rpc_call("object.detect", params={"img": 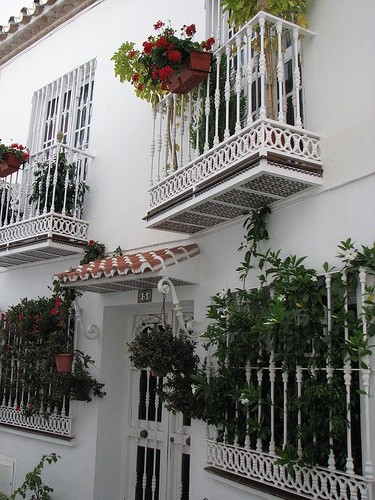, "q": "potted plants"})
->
[111,18,215,97]
[125,295,173,379]
[28,334,104,403]
[0,138,32,176]
